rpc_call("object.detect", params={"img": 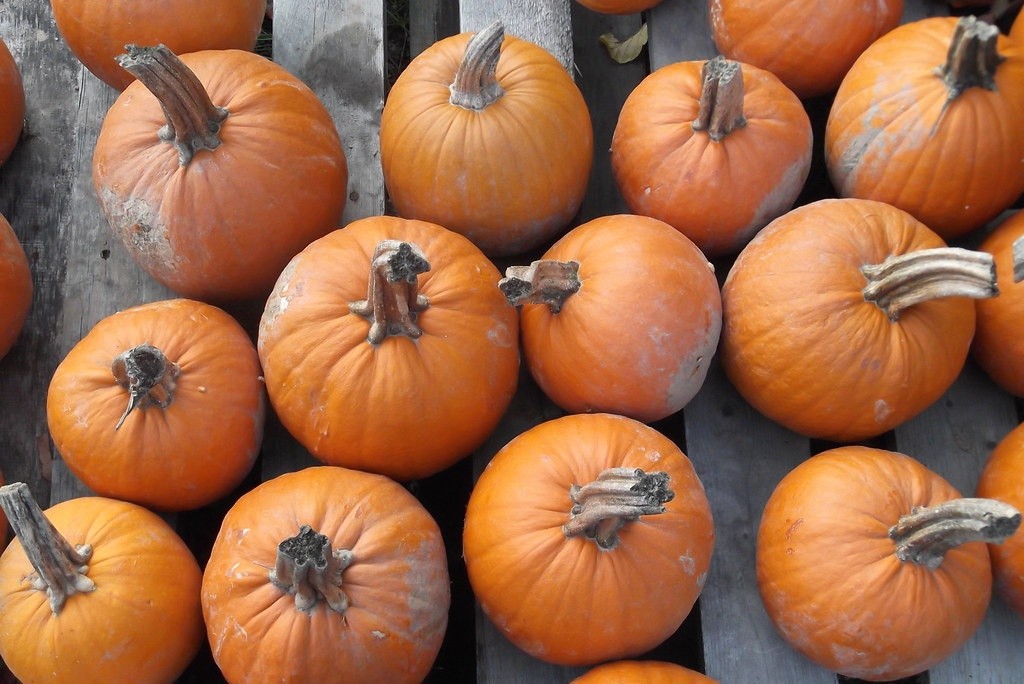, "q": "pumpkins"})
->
[0,0,1024,684]
